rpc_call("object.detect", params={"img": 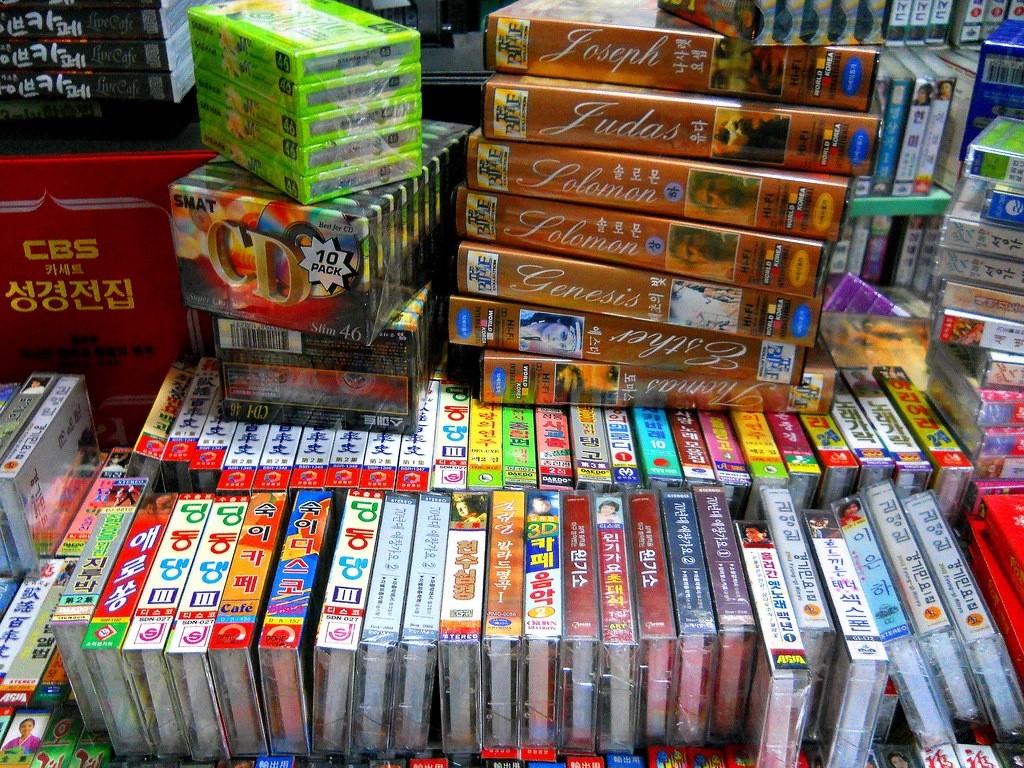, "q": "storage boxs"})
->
[0,0,1024,768]
